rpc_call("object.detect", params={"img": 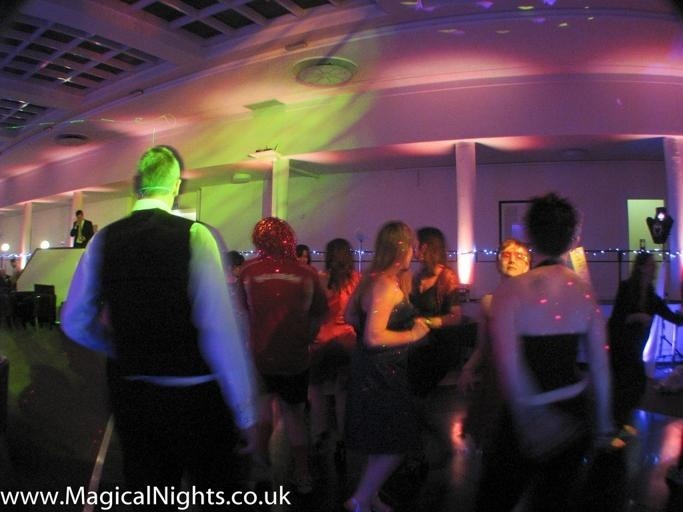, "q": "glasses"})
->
[499,252,522,261]
